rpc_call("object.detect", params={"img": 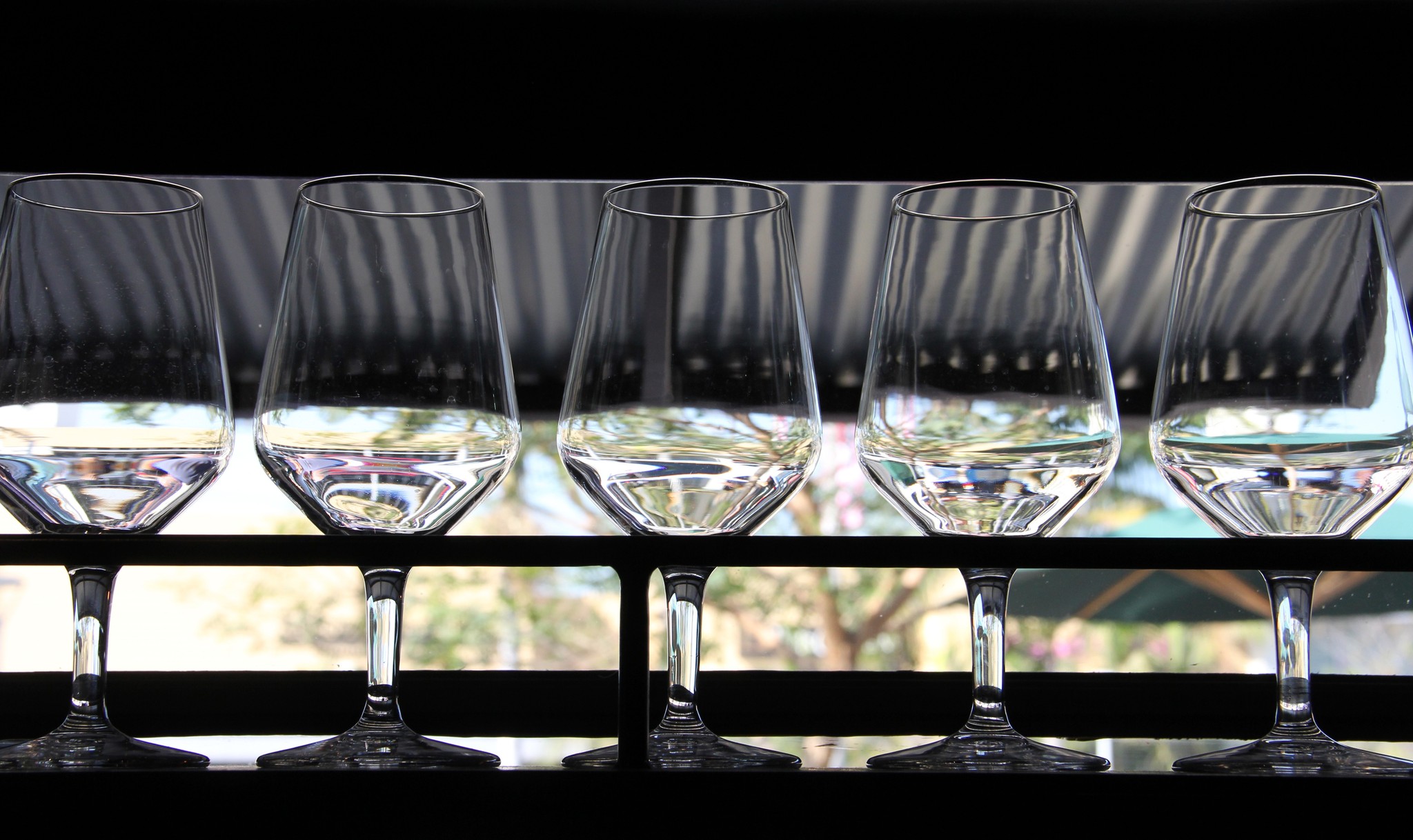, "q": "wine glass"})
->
[556,177,822,772]
[1148,171,1413,777]
[252,174,523,773]
[0,170,233,772]
[851,176,1121,773]
